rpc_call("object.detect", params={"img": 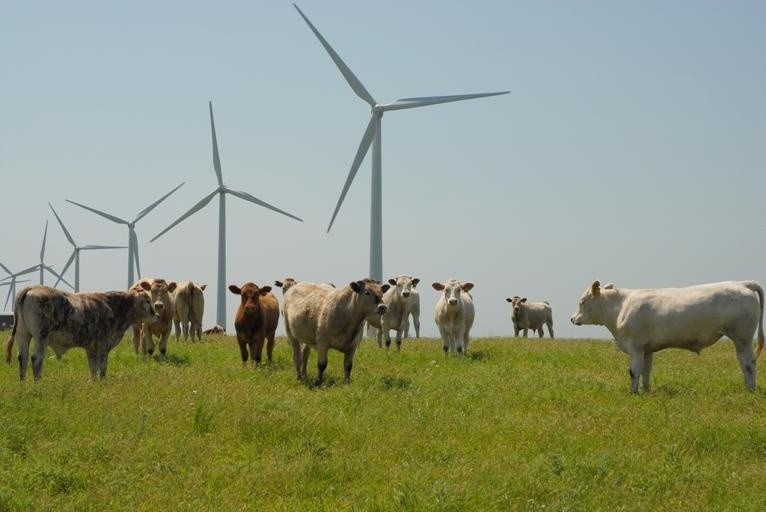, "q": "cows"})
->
[432,277,475,359]
[127,277,177,364]
[274,275,421,390]
[5,283,160,384]
[228,283,280,368]
[202,325,226,335]
[170,280,208,345]
[506,296,553,338]
[571,281,765,394]
